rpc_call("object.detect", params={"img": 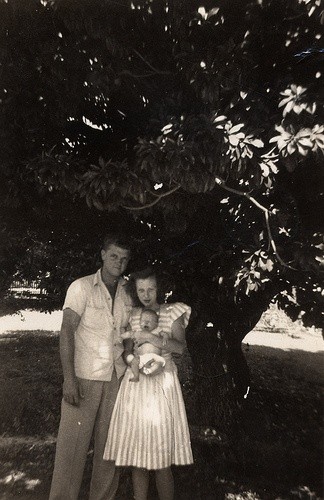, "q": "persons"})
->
[117,310,168,381]
[48,234,136,500]
[103,265,193,500]
